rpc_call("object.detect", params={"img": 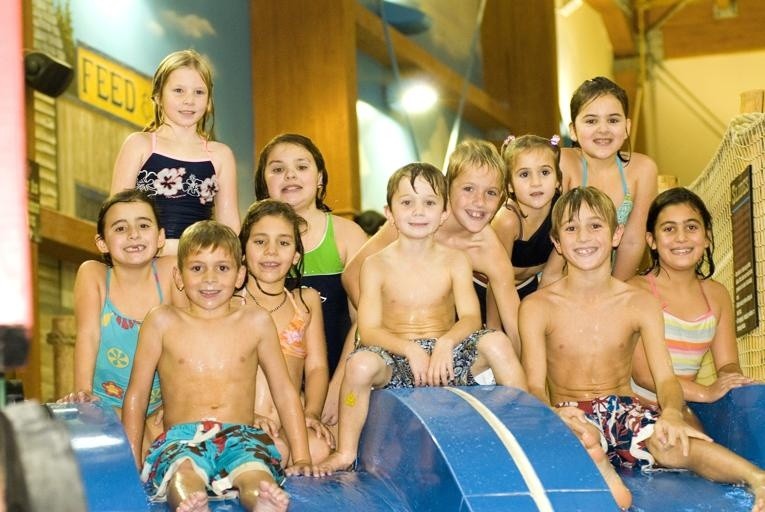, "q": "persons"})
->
[56,192,190,471]
[119,220,331,512]
[533,75,659,294]
[110,52,243,257]
[618,187,764,410]
[313,133,563,472]
[231,133,389,473]
[516,187,765,512]
